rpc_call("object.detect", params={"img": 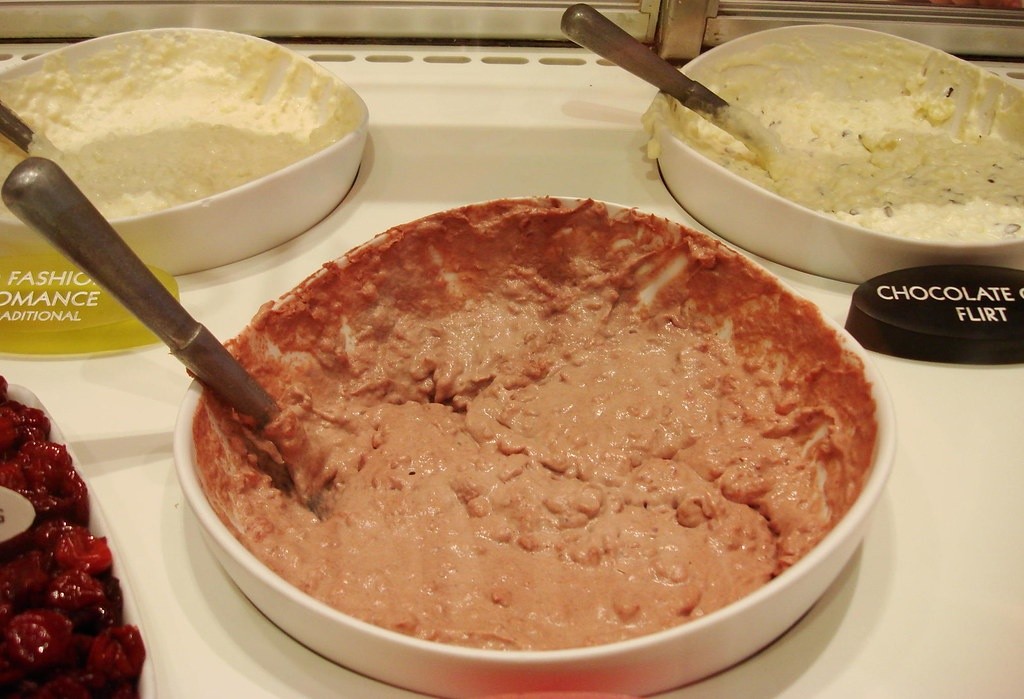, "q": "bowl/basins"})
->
[0,27,369,275]
[170,197,896,699]
[655,24,1024,284]
